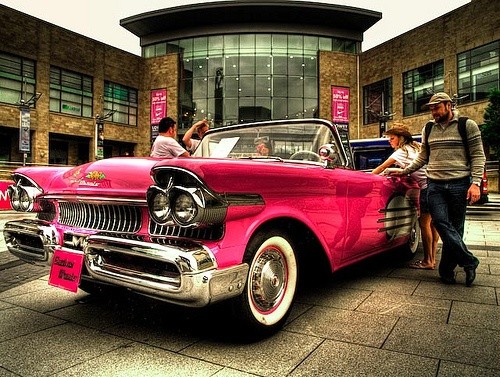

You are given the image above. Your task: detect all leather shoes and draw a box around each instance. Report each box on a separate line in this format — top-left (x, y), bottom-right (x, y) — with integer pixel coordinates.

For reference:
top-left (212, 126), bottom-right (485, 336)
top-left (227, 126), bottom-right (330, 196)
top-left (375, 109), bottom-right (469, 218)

top-left (465, 258), bottom-right (480, 286)
top-left (439, 275), bottom-right (457, 284)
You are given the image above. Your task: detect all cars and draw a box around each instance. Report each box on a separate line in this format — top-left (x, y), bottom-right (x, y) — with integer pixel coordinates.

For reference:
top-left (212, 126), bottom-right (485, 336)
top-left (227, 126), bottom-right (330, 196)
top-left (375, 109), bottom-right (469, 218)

top-left (3, 116), bottom-right (431, 336)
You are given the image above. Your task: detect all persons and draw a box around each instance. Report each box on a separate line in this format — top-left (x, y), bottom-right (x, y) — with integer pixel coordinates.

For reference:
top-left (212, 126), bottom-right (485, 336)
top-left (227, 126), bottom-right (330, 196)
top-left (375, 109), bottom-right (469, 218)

top-left (151, 117), bottom-right (192, 161)
top-left (372, 125), bottom-right (441, 270)
top-left (182, 120), bottom-right (213, 159)
top-left (382, 93), bottom-right (486, 286)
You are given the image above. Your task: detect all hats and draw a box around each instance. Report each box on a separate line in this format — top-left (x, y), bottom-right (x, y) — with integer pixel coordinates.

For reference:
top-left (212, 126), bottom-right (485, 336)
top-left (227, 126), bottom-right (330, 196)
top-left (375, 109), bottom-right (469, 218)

top-left (421, 92), bottom-right (452, 108)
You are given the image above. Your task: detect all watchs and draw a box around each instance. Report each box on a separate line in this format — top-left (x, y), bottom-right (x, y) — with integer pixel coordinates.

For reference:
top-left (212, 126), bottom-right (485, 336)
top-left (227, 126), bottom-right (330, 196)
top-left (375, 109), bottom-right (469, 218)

top-left (472, 181), bottom-right (481, 187)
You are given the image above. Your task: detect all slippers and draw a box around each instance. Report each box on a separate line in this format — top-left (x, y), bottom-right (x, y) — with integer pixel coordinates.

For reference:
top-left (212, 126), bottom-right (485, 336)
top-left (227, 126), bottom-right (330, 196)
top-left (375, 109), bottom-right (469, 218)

top-left (409, 259), bottom-right (437, 270)
